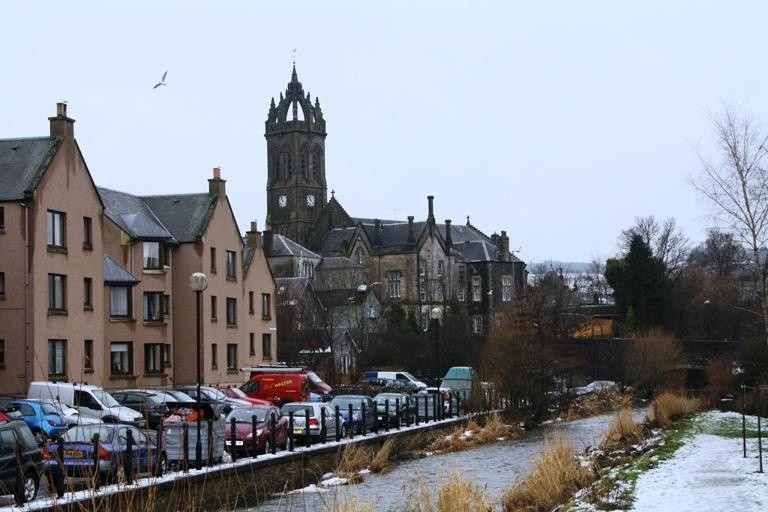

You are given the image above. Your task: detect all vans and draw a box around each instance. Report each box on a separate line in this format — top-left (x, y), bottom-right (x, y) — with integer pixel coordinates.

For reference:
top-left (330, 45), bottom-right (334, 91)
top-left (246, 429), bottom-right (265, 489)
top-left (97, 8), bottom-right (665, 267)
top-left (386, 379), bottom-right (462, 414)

top-left (364, 371), bottom-right (426, 391)
top-left (440, 366), bottom-right (483, 411)
top-left (240, 364), bottom-right (332, 403)
top-left (27, 382), bottom-right (145, 429)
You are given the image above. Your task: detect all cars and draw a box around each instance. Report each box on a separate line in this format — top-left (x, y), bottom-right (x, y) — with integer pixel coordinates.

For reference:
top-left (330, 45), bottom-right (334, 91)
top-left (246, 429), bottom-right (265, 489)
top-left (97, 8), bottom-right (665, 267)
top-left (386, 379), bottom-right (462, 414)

top-left (1, 405), bottom-right (49, 502)
top-left (111, 387), bottom-right (271, 428)
top-left (572, 381), bottom-right (613, 395)
top-left (330, 395), bottom-right (378, 435)
top-left (41, 399), bottom-right (103, 425)
top-left (2, 398), bottom-right (69, 446)
top-left (41, 423), bottom-right (166, 491)
top-left (281, 401), bottom-right (348, 443)
top-left (223, 404), bottom-right (293, 456)
top-left (373, 393), bottom-right (416, 429)
top-left (417, 388), bottom-right (464, 418)
top-left (481, 381), bottom-right (500, 409)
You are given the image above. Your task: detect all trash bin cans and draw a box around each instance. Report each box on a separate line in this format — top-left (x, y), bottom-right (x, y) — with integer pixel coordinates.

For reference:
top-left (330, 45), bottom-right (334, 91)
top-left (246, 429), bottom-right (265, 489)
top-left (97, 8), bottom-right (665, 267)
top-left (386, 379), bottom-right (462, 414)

top-left (163, 401), bottom-right (226, 466)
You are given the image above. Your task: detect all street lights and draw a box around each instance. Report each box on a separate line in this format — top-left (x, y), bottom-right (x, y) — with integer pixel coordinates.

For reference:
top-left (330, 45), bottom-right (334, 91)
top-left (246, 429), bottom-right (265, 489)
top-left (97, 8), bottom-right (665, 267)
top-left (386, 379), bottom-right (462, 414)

top-left (188, 272), bottom-right (209, 470)
top-left (431, 305), bottom-right (442, 418)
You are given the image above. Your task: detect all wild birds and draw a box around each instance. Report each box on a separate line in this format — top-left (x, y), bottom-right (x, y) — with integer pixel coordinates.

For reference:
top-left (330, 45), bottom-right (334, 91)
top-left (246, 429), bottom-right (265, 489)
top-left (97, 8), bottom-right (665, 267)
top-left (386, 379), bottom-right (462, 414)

top-left (151, 71), bottom-right (170, 91)
